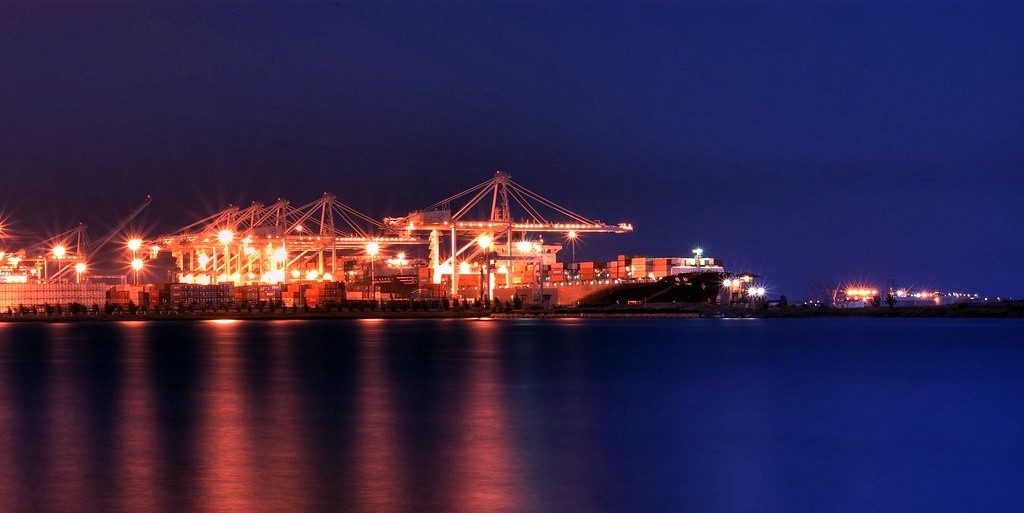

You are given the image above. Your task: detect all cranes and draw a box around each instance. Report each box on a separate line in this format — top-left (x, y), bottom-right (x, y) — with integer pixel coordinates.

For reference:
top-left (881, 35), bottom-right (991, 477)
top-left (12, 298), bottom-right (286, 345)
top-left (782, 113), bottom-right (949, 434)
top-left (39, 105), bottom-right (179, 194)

top-left (0, 168), bottom-right (634, 315)
